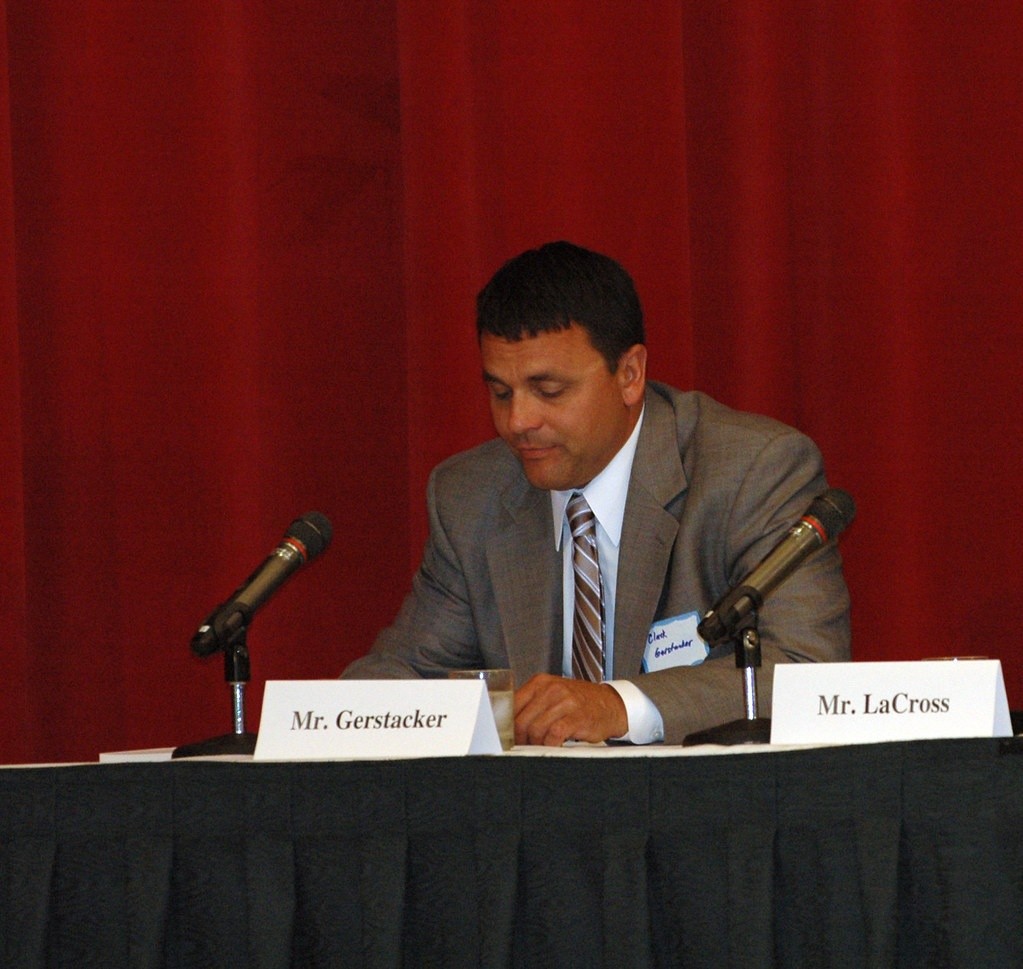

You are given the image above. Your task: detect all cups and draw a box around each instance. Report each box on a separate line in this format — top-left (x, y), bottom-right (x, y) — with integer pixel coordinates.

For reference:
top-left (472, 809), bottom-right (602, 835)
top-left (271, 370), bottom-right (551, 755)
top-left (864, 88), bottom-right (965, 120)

top-left (448, 669), bottom-right (515, 750)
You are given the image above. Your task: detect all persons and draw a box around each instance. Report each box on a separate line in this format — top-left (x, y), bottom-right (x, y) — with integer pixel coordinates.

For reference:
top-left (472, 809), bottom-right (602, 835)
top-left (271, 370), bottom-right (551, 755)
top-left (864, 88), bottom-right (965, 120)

top-left (335, 239), bottom-right (852, 751)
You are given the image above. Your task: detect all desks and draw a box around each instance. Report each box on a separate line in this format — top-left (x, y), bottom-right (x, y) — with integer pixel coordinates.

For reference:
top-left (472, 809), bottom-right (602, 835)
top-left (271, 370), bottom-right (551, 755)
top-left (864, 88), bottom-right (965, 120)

top-left (1, 735), bottom-right (1022, 969)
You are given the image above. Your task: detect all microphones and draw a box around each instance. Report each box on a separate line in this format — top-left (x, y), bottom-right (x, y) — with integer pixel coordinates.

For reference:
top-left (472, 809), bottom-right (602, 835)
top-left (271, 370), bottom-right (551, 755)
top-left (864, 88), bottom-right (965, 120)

top-left (191, 512), bottom-right (334, 658)
top-left (696, 488), bottom-right (858, 644)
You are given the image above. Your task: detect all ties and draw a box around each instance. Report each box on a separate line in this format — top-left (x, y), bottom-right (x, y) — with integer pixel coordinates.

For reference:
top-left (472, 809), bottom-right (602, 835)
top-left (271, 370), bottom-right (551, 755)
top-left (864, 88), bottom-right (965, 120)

top-left (565, 492), bottom-right (608, 685)
